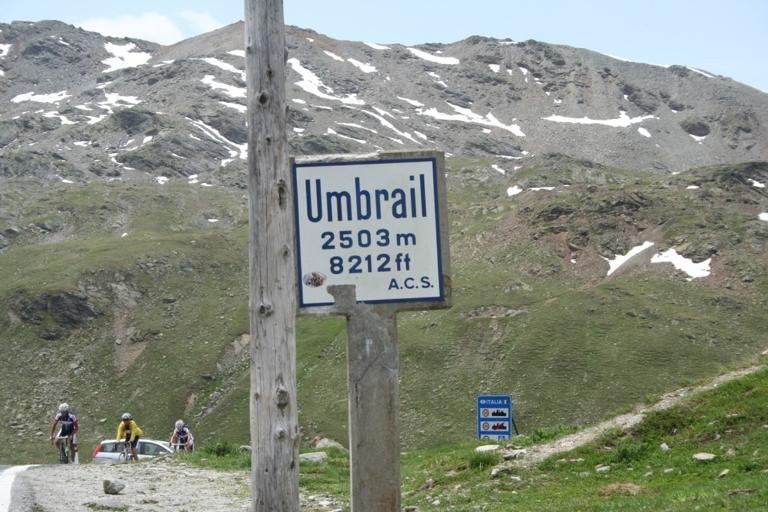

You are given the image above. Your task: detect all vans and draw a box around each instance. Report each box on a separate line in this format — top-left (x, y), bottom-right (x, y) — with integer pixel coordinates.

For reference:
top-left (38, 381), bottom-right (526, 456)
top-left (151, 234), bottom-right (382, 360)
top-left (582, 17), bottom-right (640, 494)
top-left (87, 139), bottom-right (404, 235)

top-left (91, 438), bottom-right (172, 468)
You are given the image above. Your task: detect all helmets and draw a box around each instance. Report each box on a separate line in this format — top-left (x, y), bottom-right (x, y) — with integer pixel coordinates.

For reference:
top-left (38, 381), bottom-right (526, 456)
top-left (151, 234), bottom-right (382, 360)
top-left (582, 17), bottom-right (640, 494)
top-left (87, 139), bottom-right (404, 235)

top-left (175, 419), bottom-right (183, 432)
top-left (59, 402), bottom-right (69, 412)
top-left (121, 412), bottom-right (132, 420)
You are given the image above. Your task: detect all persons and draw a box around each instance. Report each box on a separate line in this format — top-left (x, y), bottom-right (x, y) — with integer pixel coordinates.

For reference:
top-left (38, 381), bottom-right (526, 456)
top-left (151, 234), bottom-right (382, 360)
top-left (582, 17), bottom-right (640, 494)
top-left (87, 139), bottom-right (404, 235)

top-left (171, 419), bottom-right (194, 453)
top-left (115, 412), bottom-right (144, 463)
top-left (50, 403), bottom-right (79, 462)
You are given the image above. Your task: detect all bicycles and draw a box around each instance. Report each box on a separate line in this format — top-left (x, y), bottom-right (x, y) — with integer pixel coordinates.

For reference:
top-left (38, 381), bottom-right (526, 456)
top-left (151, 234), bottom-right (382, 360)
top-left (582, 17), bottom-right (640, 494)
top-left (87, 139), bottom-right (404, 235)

top-left (168, 442), bottom-right (192, 454)
top-left (50, 433), bottom-right (79, 464)
top-left (117, 440), bottom-right (144, 464)
